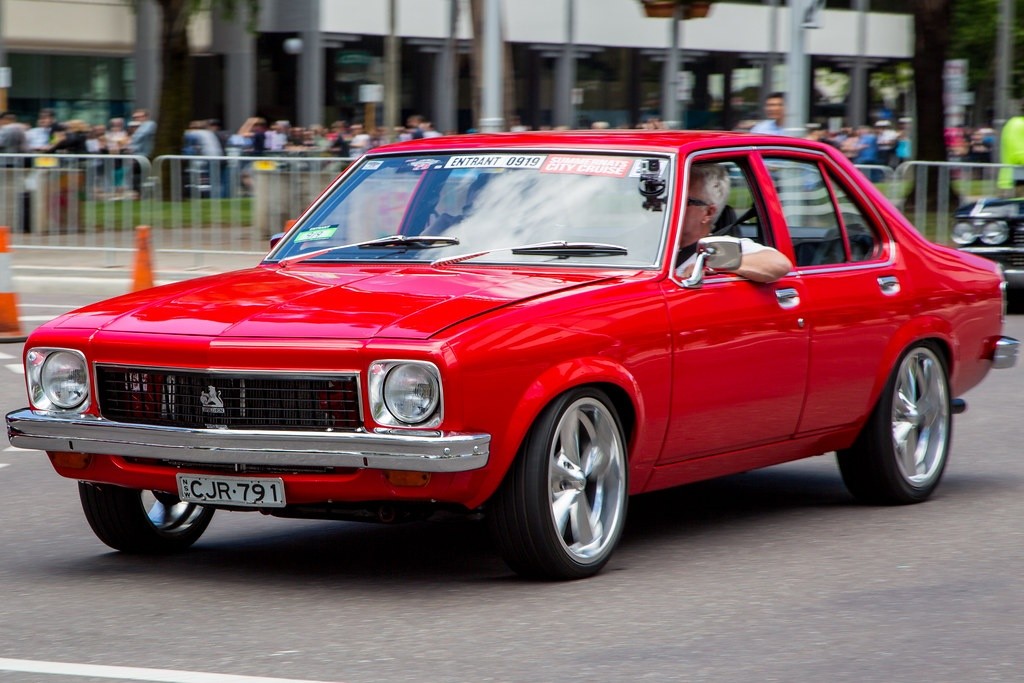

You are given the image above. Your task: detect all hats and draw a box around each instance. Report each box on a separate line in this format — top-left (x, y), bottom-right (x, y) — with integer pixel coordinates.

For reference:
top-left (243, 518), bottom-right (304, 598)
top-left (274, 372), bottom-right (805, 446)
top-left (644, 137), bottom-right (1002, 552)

top-left (898, 116), bottom-right (914, 123)
top-left (874, 119), bottom-right (892, 127)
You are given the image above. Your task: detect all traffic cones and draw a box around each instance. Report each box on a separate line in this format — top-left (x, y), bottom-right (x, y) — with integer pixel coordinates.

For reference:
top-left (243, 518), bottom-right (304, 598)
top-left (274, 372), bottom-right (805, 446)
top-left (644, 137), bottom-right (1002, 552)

top-left (1, 224), bottom-right (30, 343)
top-left (128, 224), bottom-right (155, 292)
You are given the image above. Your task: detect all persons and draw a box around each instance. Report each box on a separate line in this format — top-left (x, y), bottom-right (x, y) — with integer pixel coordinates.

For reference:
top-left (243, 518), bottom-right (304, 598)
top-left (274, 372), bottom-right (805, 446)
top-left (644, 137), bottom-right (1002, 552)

top-left (0, 92), bottom-right (1024, 233)
top-left (669, 163), bottom-right (793, 283)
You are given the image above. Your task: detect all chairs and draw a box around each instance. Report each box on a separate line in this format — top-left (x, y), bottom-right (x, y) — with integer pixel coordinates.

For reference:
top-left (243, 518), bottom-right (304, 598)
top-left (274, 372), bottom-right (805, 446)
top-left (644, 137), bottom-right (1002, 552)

top-left (712, 205), bottom-right (750, 238)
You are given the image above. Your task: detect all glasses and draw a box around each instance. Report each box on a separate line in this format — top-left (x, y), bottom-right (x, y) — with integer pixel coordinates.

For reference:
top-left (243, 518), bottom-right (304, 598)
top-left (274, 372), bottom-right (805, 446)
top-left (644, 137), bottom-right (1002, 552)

top-left (687, 198), bottom-right (711, 207)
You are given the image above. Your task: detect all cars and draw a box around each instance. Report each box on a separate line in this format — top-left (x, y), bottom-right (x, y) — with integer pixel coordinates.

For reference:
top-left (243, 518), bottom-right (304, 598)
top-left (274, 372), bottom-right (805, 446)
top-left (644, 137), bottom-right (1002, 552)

top-left (948, 193), bottom-right (1023, 303)
top-left (4, 127), bottom-right (1008, 579)
